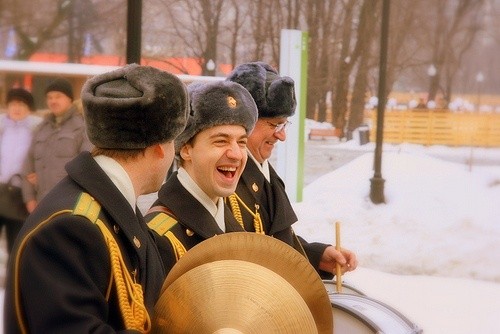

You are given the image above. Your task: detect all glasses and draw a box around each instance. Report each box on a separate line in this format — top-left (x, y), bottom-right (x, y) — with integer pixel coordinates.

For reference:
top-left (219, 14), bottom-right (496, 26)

top-left (259, 118), bottom-right (292, 133)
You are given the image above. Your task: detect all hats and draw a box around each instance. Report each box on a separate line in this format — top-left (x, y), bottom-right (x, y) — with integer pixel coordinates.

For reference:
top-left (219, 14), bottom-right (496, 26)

top-left (227, 62), bottom-right (296, 117)
top-left (6, 88), bottom-right (34, 110)
top-left (45, 76), bottom-right (74, 101)
top-left (80, 62), bottom-right (191, 151)
top-left (171, 77), bottom-right (258, 154)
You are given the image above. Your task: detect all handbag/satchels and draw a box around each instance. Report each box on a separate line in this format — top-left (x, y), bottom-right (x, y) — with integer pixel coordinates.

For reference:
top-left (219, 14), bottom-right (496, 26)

top-left (0, 173), bottom-right (31, 222)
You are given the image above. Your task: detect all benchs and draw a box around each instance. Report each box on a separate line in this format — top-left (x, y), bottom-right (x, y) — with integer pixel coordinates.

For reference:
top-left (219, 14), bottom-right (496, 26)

top-left (308, 127), bottom-right (343, 139)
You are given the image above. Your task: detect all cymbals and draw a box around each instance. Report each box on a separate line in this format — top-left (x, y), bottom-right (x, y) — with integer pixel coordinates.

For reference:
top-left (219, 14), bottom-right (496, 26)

top-left (160, 232), bottom-right (333, 334)
top-left (152, 259), bottom-right (318, 334)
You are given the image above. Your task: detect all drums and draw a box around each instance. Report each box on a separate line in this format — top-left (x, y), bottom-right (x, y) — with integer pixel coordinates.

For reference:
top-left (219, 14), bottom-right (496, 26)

top-left (322, 281), bottom-right (423, 334)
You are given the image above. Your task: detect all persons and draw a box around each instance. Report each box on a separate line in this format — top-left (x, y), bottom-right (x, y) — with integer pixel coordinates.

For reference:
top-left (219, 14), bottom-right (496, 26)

top-left (144, 81), bottom-right (259, 274)
top-left (5, 63), bottom-right (190, 334)
top-left (21, 79), bottom-right (95, 213)
top-left (1, 88), bottom-right (43, 253)
top-left (228, 62), bottom-right (357, 275)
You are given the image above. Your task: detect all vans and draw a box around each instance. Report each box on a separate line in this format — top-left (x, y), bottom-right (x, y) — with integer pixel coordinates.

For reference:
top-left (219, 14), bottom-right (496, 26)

top-left (1, 60), bottom-right (112, 116)
